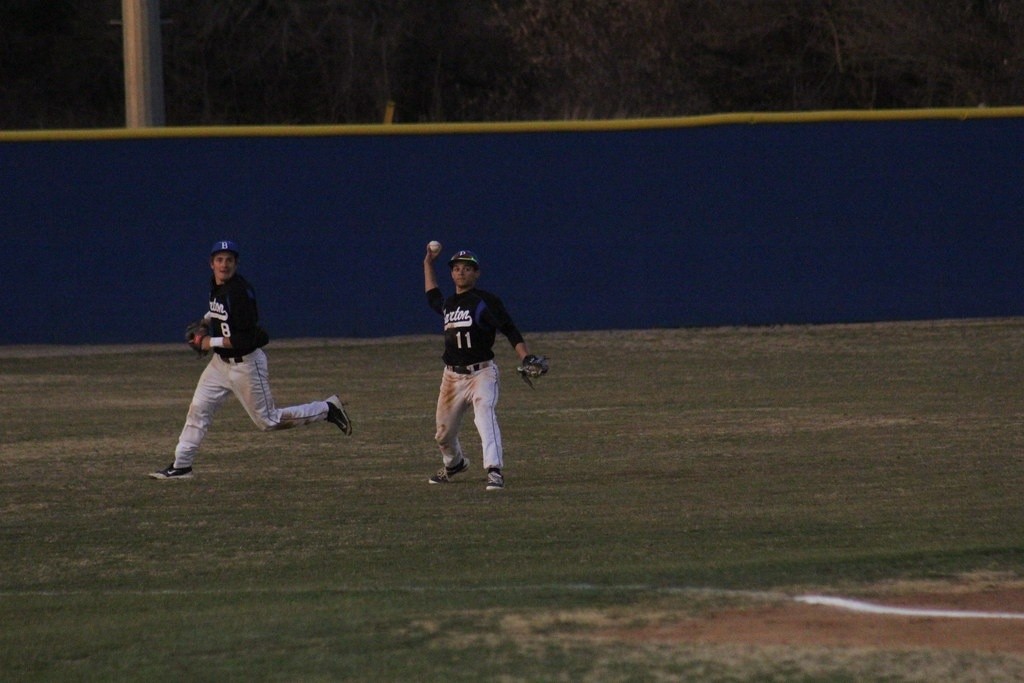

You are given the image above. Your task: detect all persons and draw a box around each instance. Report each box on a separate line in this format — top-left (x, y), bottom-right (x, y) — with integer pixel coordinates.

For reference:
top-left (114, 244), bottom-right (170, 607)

top-left (423, 241), bottom-right (550, 491)
top-left (146, 241), bottom-right (353, 478)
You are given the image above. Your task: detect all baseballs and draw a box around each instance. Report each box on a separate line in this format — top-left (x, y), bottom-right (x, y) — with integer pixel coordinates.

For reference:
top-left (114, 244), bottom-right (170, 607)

top-left (428, 240), bottom-right (441, 252)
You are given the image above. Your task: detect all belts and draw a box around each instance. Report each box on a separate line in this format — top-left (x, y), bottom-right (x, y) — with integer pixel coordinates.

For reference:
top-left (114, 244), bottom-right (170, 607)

top-left (447, 364), bottom-right (479, 374)
top-left (220, 355), bottom-right (243, 363)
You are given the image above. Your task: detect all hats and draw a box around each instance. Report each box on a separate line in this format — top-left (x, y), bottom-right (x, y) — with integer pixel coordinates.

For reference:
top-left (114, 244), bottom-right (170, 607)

top-left (448, 250), bottom-right (479, 268)
top-left (211, 241), bottom-right (239, 256)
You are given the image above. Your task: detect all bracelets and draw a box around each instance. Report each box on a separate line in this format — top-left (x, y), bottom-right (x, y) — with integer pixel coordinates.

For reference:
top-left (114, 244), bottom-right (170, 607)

top-left (209, 337), bottom-right (224, 348)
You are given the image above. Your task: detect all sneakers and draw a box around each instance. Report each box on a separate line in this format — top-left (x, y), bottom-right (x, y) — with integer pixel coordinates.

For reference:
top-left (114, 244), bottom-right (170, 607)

top-left (485, 471), bottom-right (504, 491)
top-left (325, 394), bottom-right (352, 436)
top-left (149, 463), bottom-right (193, 479)
top-left (429, 458), bottom-right (470, 483)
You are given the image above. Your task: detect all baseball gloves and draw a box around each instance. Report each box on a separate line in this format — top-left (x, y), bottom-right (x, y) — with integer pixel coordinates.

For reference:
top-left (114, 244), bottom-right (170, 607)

top-left (516, 354), bottom-right (550, 379)
top-left (184, 318), bottom-right (213, 354)
